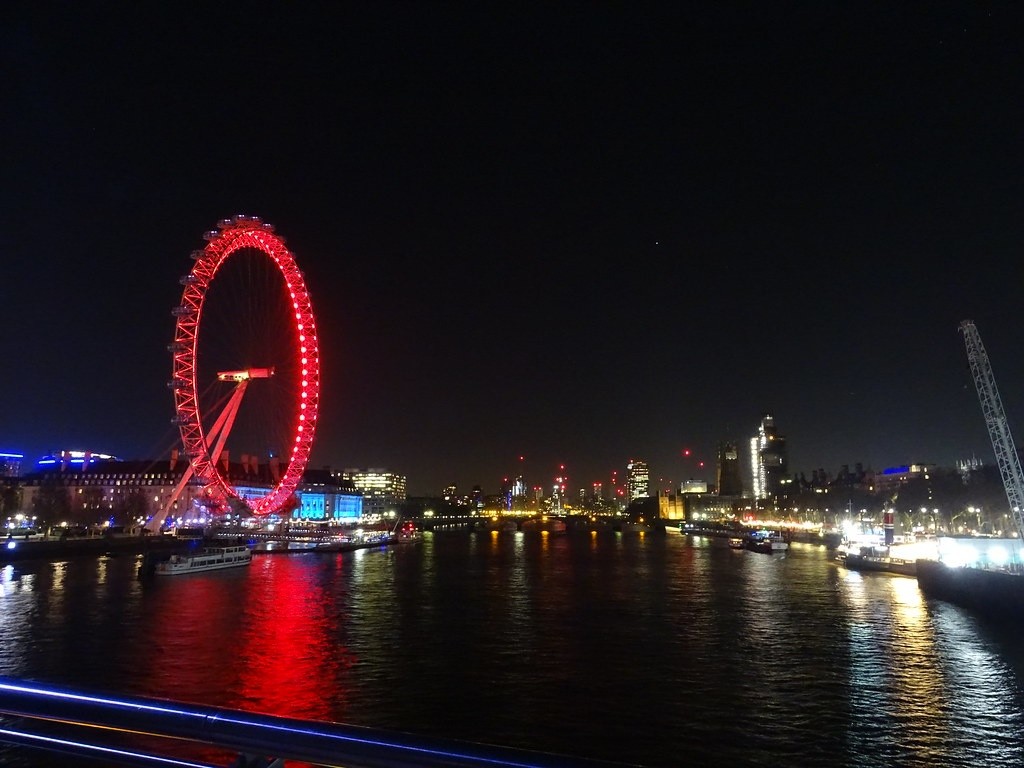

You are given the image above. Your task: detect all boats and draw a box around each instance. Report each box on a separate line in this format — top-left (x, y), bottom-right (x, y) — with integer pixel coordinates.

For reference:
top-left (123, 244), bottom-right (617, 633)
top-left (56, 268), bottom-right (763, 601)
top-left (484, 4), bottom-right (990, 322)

top-left (842, 543), bottom-right (891, 572)
top-left (725, 531), bottom-right (791, 555)
top-left (153, 545), bottom-right (253, 575)
